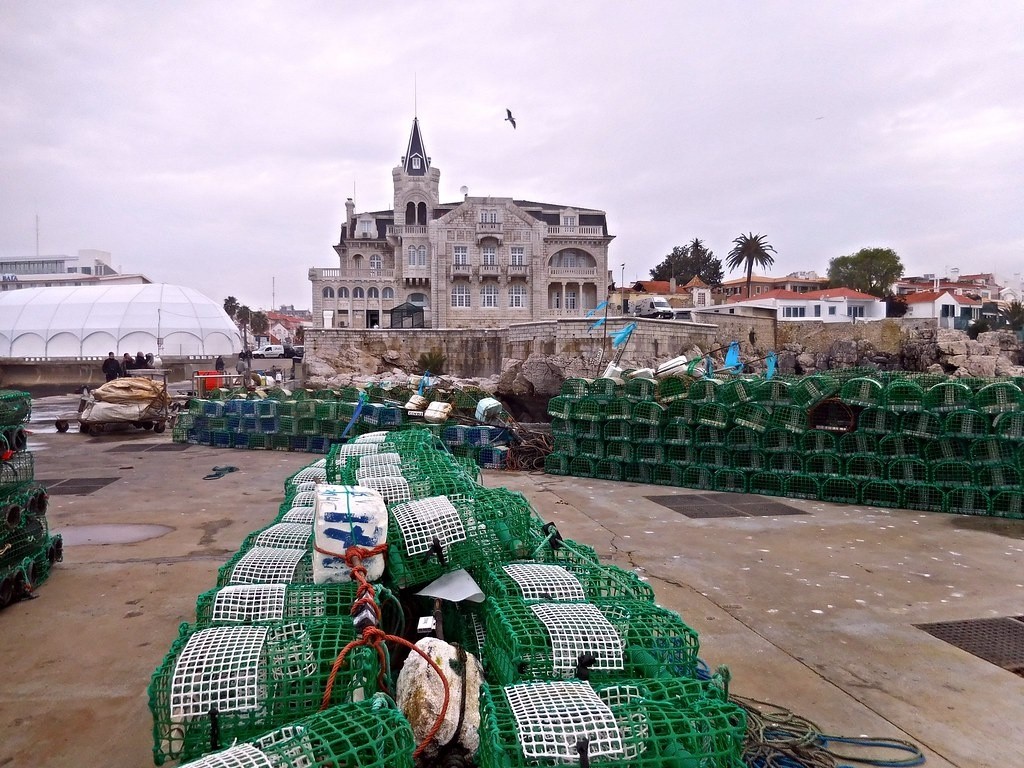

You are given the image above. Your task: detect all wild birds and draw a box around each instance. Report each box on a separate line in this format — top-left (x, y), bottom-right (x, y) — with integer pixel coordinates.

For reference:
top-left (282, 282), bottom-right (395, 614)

top-left (505, 109), bottom-right (518, 129)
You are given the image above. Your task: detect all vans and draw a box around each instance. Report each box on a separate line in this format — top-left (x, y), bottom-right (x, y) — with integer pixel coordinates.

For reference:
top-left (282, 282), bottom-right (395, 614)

top-left (634, 296), bottom-right (674, 319)
top-left (251, 344), bottom-right (285, 360)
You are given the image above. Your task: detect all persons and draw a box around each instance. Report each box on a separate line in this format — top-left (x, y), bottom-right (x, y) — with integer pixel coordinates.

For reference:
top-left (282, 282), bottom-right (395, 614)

top-left (246, 350), bottom-right (252, 360)
top-left (102, 351), bottom-right (122, 382)
top-left (135, 351), bottom-right (155, 369)
top-left (216, 355), bottom-right (225, 370)
top-left (119, 353), bottom-right (134, 377)
top-left (236, 358), bottom-right (246, 375)
top-left (239, 350), bottom-right (245, 360)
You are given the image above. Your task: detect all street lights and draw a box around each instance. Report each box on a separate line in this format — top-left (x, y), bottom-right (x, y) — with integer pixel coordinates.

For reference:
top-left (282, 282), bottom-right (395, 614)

top-left (621, 263), bottom-right (625, 316)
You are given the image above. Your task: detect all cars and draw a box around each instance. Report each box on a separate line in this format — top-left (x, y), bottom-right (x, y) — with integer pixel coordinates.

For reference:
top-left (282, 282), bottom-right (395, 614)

top-left (282, 345), bottom-right (304, 359)
